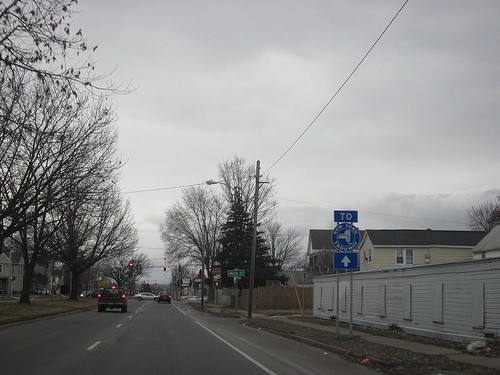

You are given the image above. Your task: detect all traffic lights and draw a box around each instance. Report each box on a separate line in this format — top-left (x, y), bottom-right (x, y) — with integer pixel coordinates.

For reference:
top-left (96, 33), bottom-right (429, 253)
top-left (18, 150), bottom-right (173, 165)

top-left (164, 265), bottom-right (166, 271)
top-left (129, 262), bottom-right (133, 270)
top-left (12, 275), bottom-right (16, 281)
top-left (136, 264), bottom-right (138, 270)
top-left (140, 264), bottom-right (142, 271)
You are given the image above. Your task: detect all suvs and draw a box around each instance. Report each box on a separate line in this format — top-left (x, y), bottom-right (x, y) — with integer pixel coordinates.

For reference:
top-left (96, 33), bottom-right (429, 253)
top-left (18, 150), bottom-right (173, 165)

top-left (97, 287), bottom-right (128, 313)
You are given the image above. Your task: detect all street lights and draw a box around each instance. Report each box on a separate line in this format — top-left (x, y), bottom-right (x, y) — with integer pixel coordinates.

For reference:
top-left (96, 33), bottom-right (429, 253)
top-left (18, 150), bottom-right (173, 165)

top-left (204, 179), bottom-right (254, 321)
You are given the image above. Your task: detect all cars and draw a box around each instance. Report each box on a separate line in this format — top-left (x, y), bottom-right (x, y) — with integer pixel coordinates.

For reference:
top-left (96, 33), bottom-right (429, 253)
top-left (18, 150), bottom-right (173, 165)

top-left (81, 289), bottom-right (99, 299)
top-left (135, 292), bottom-right (159, 302)
top-left (157, 292), bottom-right (172, 304)
